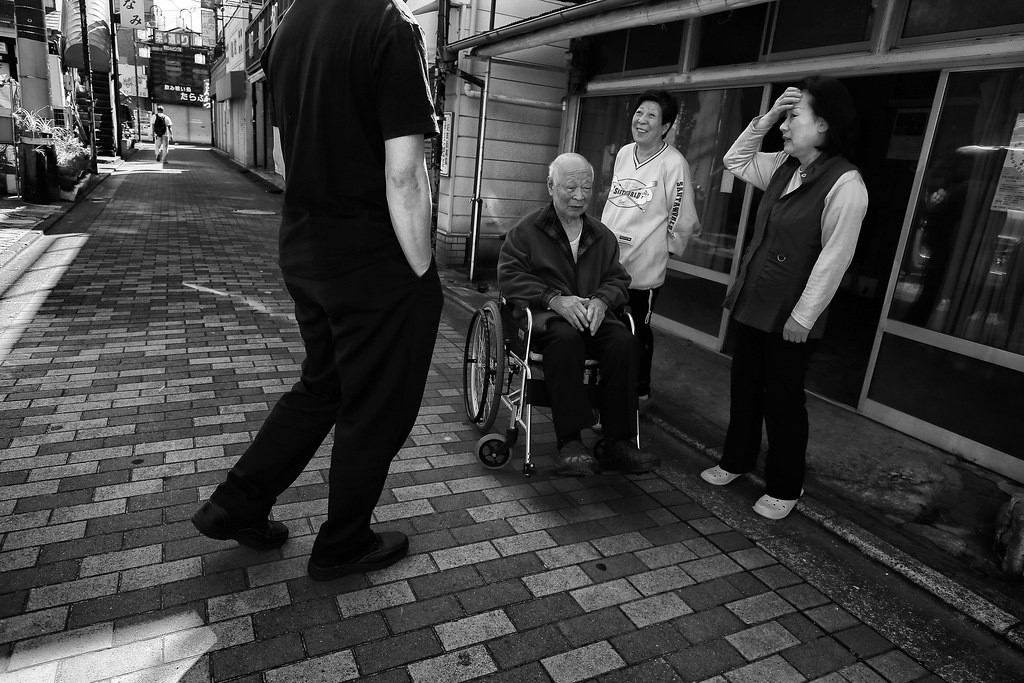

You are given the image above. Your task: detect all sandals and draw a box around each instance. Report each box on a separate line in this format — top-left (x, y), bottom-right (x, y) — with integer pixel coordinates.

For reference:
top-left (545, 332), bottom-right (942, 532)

top-left (752, 488), bottom-right (804, 519)
top-left (701, 465), bottom-right (743, 485)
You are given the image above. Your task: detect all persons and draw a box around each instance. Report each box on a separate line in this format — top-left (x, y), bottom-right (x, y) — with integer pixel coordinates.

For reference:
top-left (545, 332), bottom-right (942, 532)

top-left (700, 76), bottom-right (868, 519)
top-left (192, 1), bottom-right (445, 580)
top-left (602, 89), bottom-right (702, 416)
top-left (497, 152), bottom-right (652, 476)
top-left (151, 106), bottom-right (173, 163)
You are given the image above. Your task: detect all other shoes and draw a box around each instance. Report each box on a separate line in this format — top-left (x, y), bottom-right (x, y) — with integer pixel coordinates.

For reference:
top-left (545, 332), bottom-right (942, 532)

top-left (603, 439), bottom-right (660, 467)
top-left (155, 153), bottom-right (160, 162)
top-left (163, 162), bottom-right (168, 164)
top-left (557, 440), bottom-right (599, 478)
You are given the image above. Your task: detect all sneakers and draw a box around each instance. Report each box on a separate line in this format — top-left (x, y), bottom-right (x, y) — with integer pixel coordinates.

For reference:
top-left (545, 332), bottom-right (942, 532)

top-left (191, 498), bottom-right (288, 550)
top-left (307, 528), bottom-right (409, 583)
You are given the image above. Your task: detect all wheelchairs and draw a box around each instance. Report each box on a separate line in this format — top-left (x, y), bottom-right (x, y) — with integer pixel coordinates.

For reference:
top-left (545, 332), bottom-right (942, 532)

top-left (462, 232), bottom-right (642, 480)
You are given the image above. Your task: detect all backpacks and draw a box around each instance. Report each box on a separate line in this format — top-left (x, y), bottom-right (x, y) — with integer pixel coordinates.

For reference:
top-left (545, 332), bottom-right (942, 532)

top-left (154, 114), bottom-right (167, 137)
